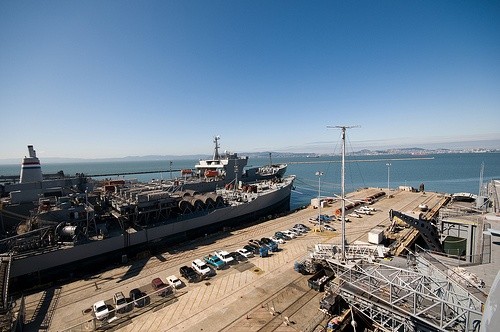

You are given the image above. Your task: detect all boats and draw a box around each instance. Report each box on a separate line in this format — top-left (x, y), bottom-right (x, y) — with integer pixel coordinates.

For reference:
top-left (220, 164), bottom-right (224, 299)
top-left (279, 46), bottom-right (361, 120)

top-left (0, 134), bottom-right (296, 280)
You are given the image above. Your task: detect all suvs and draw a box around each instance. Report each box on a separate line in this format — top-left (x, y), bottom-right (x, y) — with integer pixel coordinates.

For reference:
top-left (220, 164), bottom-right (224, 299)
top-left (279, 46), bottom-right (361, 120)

top-left (113, 291), bottom-right (128, 314)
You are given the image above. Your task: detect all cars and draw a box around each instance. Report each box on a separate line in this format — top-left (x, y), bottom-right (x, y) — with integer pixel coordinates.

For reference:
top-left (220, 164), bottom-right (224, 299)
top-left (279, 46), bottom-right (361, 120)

top-left (166, 275), bottom-right (183, 289)
top-left (151, 277), bottom-right (168, 297)
top-left (128, 289), bottom-right (145, 309)
top-left (92, 300), bottom-right (110, 320)
top-left (180, 205), bottom-right (377, 284)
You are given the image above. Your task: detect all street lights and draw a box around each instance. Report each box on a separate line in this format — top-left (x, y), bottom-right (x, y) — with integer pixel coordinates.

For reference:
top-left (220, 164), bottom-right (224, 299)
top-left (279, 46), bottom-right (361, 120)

top-left (314, 171), bottom-right (324, 228)
top-left (386, 163), bottom-right (393, 197)
